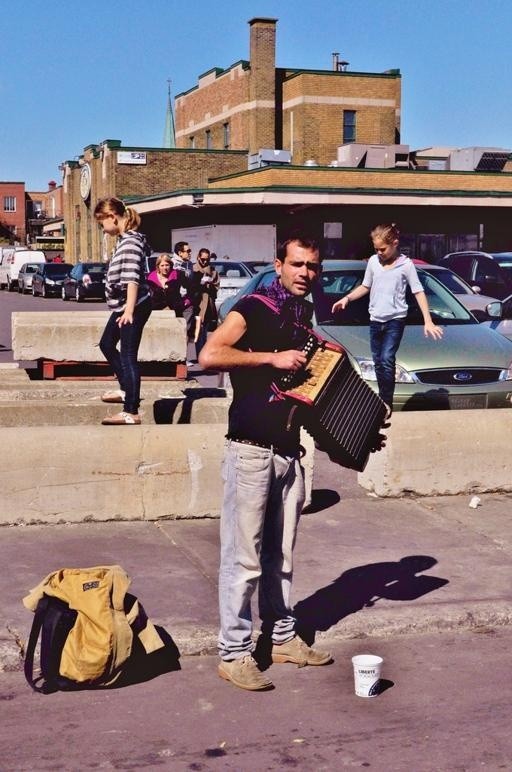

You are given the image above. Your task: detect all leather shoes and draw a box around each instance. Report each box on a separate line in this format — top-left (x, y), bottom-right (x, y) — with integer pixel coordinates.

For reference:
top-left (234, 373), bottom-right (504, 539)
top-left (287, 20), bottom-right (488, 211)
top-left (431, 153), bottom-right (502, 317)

top-left (101, 389), bottom-right (126, 403)
top-left (100, 412), bottom-right (143, 424)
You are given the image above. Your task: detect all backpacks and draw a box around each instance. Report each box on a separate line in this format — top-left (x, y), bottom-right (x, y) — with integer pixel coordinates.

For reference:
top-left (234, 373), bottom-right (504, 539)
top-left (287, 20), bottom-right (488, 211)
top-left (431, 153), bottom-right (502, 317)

top-left (21, 562), bottom-right (166, 695)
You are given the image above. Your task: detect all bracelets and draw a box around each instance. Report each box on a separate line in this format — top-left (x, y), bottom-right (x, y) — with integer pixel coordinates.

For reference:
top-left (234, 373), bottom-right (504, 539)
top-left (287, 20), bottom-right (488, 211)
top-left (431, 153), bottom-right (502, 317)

top-left (344, 295), bottom-right (351, 305)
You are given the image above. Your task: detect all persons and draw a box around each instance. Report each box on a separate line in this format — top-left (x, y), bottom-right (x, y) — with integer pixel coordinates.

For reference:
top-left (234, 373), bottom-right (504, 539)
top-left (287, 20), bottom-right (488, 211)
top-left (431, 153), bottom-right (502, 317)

top-left (146, 241), bottom-right (230, 368)
top-left (91, 195), bottom-right (153, 425)
top-left (331, 221), bottom-right (444, 418)
top-left (197, 235), bottom-right (334, 693)
top-left (52, 254), bottom-right (61, 264)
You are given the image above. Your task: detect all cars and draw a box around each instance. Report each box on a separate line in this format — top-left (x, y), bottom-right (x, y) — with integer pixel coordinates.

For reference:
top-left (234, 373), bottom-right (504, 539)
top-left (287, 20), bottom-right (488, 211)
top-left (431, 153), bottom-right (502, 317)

top-left (218, 257), bottom-right (511, 408)
top-left (15, 262), bottom-right (112, 302)
top-left (208, 260), bottom-right (270, 315)
top-left (415, 249), bottom-right (511, 352)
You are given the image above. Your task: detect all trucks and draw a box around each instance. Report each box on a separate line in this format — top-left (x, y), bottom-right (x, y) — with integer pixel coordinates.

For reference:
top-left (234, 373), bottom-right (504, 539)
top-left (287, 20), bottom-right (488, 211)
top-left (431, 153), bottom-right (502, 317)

top-left (0, 249), bottom-right (47, 290)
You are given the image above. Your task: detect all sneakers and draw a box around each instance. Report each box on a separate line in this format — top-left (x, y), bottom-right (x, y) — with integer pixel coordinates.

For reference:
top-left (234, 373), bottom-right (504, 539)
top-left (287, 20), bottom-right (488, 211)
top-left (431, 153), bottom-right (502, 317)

top-left (216, 655), bottom-right (274, 693)
top-left (269, 635), bottom-right (332, 666)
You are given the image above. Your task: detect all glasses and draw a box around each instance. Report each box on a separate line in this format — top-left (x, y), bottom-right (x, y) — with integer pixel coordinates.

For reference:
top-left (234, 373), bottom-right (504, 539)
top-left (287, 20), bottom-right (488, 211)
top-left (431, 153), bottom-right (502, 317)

top-left (200, 257), bottom-right (211, 262)
top-left (181, 248), bottom-right (192, 253)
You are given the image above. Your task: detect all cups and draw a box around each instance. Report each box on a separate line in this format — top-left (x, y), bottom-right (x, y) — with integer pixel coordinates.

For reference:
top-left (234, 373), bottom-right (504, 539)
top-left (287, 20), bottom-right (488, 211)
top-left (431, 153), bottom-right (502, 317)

top-left (352, 654), bottom-right (384, 699)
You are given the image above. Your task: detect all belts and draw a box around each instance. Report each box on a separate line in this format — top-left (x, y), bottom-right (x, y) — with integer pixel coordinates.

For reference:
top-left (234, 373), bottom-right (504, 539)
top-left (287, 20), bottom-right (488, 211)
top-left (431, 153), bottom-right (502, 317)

top-left (226, 438), bottom-right (301, 453)
